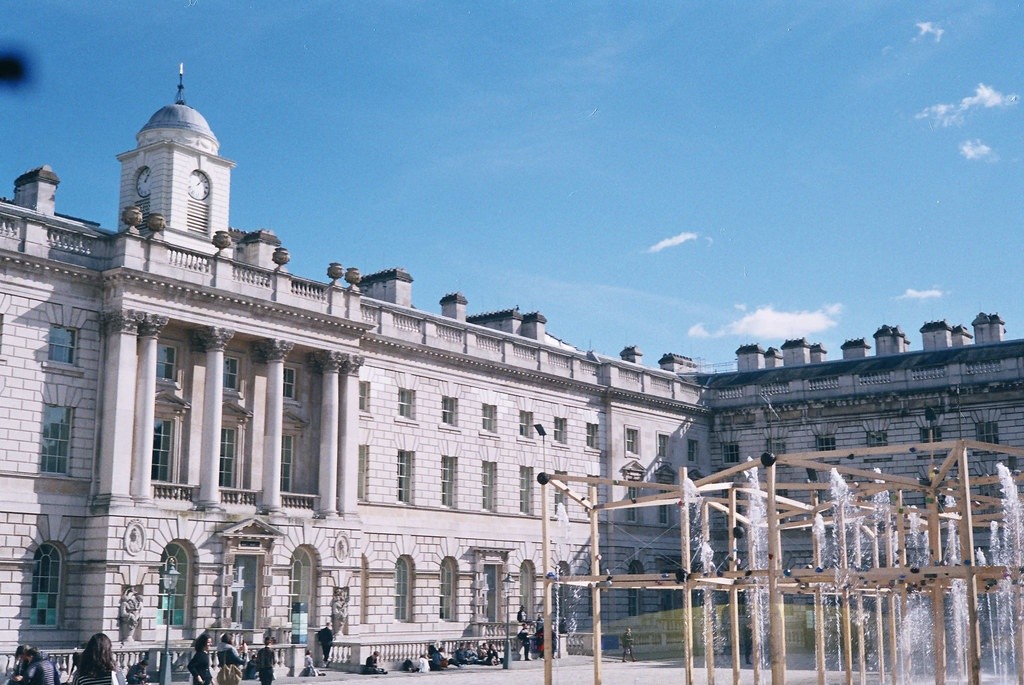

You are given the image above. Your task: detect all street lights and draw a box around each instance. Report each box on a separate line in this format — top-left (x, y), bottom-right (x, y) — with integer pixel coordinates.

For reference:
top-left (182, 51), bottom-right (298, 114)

top-left (501, 571), bottom-right (516, 669)
top-left (159, 555), bottom-right (180, 685)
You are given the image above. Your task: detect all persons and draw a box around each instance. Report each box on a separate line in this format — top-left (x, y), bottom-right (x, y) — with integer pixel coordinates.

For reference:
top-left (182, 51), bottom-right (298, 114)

top-left (318, 621), bottom-right (333, 668)
top-left (365, 650), bottom-right (388, 674)
top-left (4, 632), bottom-right (278, 685)
top-left (622, 627), bottom-right (637, 663)
top-left (517, 605), bottom-right (568, 661)
top-left (743, 622), bottom-right (753, 665)
top-left (409, 642), bottom-right (505, 674)
top-left (304, 650), bottom-right (326, 677)
